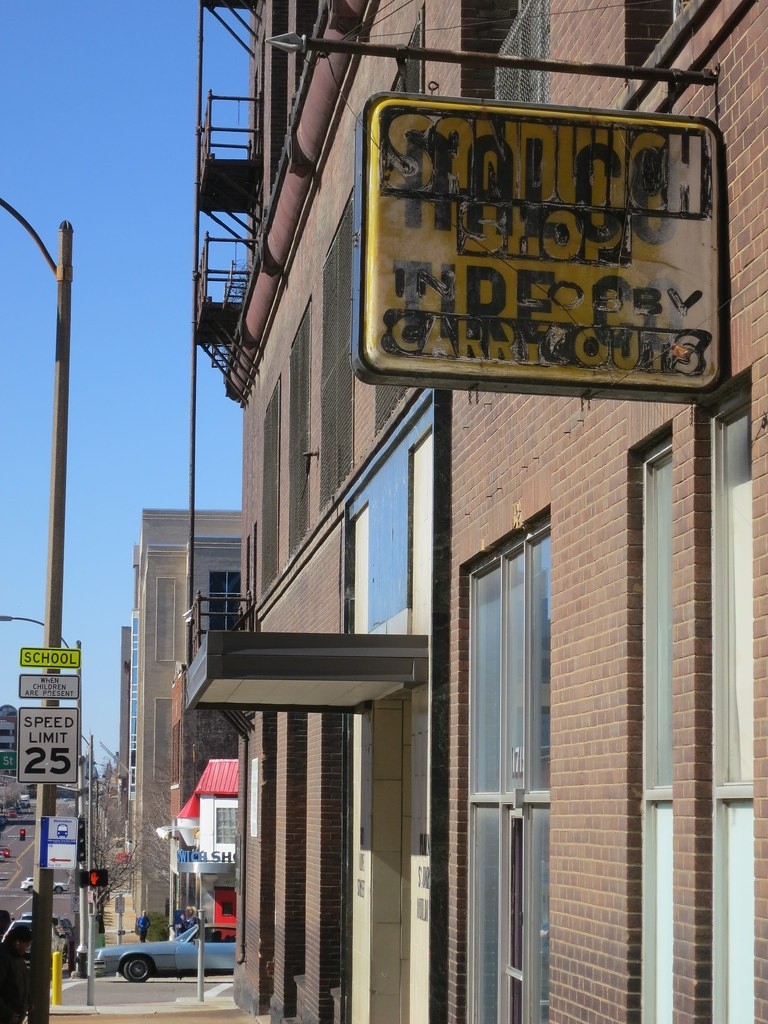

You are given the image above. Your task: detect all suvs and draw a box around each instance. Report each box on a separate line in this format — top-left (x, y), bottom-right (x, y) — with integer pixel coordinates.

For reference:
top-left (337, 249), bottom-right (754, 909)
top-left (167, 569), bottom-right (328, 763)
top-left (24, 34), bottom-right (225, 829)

top-left (18, 912), bottom-right (73, 947)
top-left (1, 919), bottom-right (67, 969)
top-left (20, 877), bottom-right (69, 893)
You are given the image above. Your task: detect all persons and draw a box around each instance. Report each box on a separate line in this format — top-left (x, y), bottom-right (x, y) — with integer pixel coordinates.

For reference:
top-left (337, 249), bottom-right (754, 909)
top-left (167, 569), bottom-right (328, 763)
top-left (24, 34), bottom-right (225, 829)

top-left (179, 906), bottom-right (196, 934)
top-left (137, 910), bottom-right (150, 943)
top-left (0, 909), bottom-right (31, 1024)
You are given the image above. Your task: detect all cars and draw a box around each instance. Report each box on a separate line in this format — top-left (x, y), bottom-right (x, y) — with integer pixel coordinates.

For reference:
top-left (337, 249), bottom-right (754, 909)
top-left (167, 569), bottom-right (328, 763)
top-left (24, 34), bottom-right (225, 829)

top-left (0, 847), bottom-right (10, 862)
top-left (94, 923), bottom-right (238, 983)
top-left (7, 805), bottom-right (19, 818)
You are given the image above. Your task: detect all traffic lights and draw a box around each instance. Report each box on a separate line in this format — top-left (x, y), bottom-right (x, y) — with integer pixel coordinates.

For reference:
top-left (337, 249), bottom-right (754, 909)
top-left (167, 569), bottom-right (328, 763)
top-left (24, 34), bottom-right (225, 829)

top-left (90, 869), bottom-right (108, 887)
top-left (20, 828), bottom-right (25, 841)
top-left (78, 817), bottom-right (87, 861)
top-left (80, 869), bottom-right (90, 888)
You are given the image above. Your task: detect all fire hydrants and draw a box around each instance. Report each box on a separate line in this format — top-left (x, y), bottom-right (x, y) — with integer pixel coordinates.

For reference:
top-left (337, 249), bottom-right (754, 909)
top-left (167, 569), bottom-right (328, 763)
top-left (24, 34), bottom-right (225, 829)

top-left (74, 953), bottom-right (87, 979)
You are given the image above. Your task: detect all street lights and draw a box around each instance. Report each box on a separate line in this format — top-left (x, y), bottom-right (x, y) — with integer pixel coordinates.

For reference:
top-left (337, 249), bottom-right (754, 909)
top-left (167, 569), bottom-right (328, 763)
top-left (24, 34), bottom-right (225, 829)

top-left (0, 615), bottom-right (87, 952)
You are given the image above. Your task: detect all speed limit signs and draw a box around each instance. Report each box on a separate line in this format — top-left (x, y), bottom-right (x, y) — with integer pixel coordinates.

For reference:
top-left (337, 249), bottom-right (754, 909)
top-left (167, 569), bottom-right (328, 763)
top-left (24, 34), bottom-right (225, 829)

top-left (16, 706), bottom-right (79, 784)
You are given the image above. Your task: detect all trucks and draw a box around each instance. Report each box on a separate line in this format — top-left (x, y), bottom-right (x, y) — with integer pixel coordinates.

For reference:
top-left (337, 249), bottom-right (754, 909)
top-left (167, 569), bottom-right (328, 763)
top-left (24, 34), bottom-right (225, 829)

top-left (20, 794), bottom-right (30, 806)
